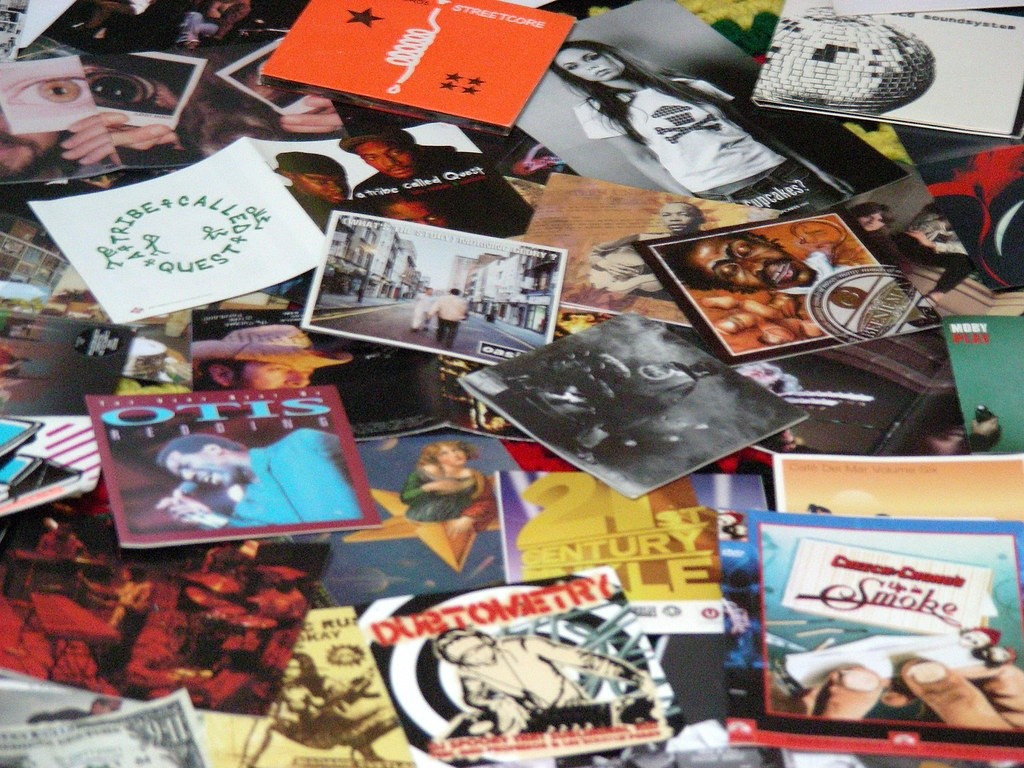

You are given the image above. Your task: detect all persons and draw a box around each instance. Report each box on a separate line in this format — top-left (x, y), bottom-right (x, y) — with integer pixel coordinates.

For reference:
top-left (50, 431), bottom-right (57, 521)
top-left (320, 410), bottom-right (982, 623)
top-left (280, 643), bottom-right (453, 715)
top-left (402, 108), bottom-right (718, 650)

top-left (0, 0), bottom-right (1024, 768)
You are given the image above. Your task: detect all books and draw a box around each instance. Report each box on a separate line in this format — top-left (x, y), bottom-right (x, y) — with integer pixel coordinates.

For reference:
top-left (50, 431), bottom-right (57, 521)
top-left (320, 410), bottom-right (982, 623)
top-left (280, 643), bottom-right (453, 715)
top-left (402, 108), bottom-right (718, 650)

top-left (257, 1), bottom-right (575, 136)
top-left (86, 385), bottom-right (384, 550)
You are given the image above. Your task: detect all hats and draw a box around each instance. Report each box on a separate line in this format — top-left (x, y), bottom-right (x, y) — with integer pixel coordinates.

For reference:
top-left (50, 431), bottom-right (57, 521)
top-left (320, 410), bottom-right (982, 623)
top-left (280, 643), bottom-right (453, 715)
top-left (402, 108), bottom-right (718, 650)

top-left (273, 152), bottom-right (346, 176)
top-left (338, 126), bottom-right (413, 153)
